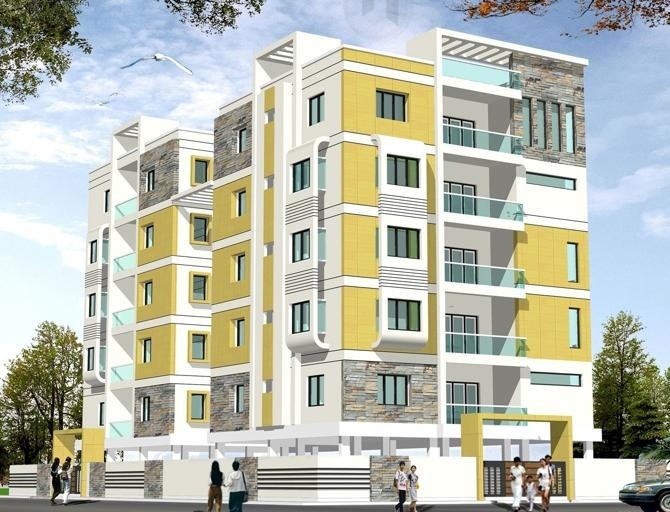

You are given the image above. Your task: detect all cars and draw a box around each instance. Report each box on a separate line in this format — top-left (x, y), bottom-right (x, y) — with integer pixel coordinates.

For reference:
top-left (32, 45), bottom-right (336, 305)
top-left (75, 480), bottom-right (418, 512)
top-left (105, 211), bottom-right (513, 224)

top-left (619, 460), bottom-right (670, 511)
